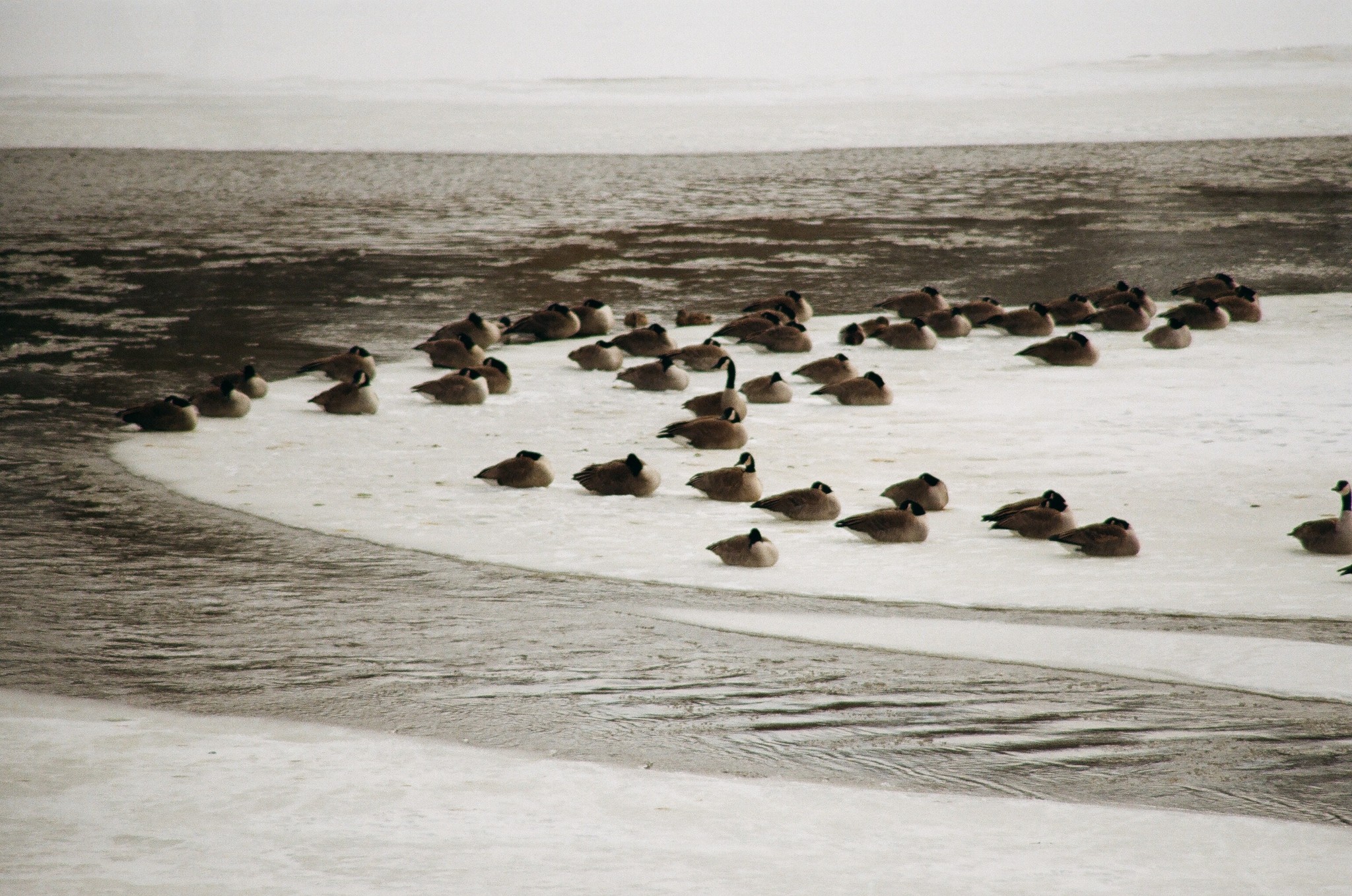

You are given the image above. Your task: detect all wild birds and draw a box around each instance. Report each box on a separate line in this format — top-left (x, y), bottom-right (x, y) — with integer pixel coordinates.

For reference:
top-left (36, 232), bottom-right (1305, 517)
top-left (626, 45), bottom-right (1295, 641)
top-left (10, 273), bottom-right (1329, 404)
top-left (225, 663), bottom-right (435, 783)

top-left (409, 356), bottom-right (513, 405)
top-left (1285, 479), bottom-right (1352, 578)
top-left (1050, 514), bottom-right (1149, 564)
top-left (412, 297), bottom-right (617, 370)
top-left (295, 344), bottom-right (377, 385)
top-left (473, 449), bottom-right (555, 489)
top-left (704, 527), bottom-right (780, 568)
top-left (981, 487), bottom-right (1079, 542)
top-left (306, 369), bottom-right (380, 416)
top-left (596, 272), bottom-right (1262, 520)
top-left (190, 363), bottom-right (269, 419)
top-left (115, 396), bottom-right (199, 433)
top-left (572, 451), bottom-right (662, 497)
top-left (834, 500), bottom-right (929, 544)
